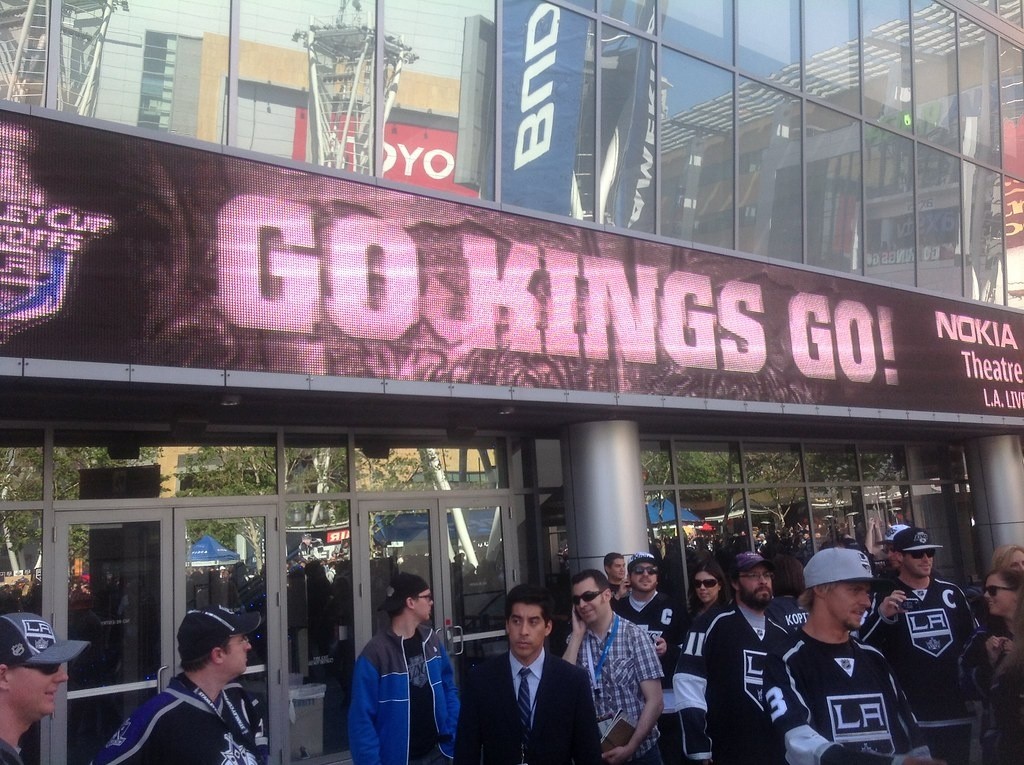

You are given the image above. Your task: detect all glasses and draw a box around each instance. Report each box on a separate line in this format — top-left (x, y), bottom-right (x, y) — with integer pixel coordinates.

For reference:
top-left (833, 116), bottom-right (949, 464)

top-left (38, 663), bottom-right (61, 674)
top-left (741, 571), bottom-right (775, 580)
top-left (910, 548), bottom-right (935, 558)
top-left (418, 592), bottom-right (432, 600)
top-left (984, 585), bottom-right (1014, 595)
top-left (692, 579), bottom-right (719, 589)
top-left (225, 634), bottom-right (249, 645)
top-left (573, 586), bottom-right (607, 605)
top-left (633, 566), bottom-right (658, 574)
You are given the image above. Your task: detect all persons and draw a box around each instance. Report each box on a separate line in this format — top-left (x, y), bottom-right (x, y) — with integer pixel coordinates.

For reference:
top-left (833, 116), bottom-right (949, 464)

top-left (191, 542), bottom-right (349, 587)
top-left (89, 605), bottom-right (273, 765)
top-left (453, 584), bottom-right (606, 765)
top-left (6, 573), bottom-right (90, 596)
top-left (347, 572), bottom-right (458, 765)
top-left (0, 611), bottom-right (89, 765)
top-left (651, 520), bottom-right (844, 559)
top-left (560, 524), bottom-right (1024, 765)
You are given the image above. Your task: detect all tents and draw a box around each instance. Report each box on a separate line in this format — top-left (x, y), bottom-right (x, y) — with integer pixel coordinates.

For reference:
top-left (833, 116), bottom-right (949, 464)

top-left (185, 534), bottom-right (241, 567)
top-left (644, 496), bottom-right (700, 526)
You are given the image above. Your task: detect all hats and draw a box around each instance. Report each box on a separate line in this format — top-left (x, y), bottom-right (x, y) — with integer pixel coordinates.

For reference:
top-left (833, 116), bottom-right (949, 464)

top-left (804, 546), bottom-right (895, 594)
top-left (894, 526), bottom-right (944, 551)
top-left (628, 550), bottom-right (656, 574)
top-left (177, 605), bottom-right (261, 662)
top-left (0, 612), bottom-right (91, 666)
top-left (377, 572), bottom-right (429, 611)
top-left (728, 552), bottom-right (775, 580)
top-left (875, 524), bottom-right (911, 544)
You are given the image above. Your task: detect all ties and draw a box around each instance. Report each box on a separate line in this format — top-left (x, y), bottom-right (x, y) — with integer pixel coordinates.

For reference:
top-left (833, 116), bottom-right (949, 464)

top-left (518, 668), bottom-right (532, 752)
